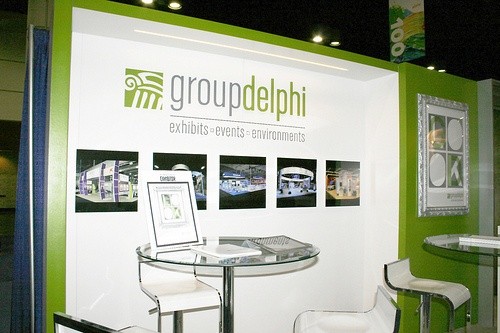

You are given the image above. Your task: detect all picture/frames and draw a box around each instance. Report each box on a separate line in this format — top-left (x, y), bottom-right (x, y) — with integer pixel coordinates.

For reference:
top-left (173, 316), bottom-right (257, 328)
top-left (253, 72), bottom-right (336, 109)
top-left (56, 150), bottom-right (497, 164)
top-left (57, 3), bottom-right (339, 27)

top-left (418, 93), bottom-right (470, 216)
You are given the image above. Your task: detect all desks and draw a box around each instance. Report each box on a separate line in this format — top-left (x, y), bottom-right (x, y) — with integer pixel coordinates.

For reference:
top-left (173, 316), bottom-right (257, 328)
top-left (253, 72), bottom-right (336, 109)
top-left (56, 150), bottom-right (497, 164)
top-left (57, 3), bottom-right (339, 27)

top-left (136, 236), bottom-right (320, 333)
top-left (424, 234), bottom-right (500, 333)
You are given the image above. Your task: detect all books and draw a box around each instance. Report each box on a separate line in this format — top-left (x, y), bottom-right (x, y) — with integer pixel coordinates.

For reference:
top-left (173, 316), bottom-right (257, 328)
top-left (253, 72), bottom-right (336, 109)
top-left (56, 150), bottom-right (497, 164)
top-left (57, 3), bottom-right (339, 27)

top-left (248, 235), bottom-right (313, 254)
top-left (459, 234), bottom-right (500, 249)
top-left (191, 244), bottom-right (262, 259)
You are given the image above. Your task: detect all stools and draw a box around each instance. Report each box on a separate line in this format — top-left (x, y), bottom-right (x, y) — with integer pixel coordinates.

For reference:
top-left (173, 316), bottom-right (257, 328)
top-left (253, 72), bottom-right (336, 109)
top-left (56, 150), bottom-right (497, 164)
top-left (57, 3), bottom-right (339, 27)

top-left (294, 286), bottom-right (401, 333)
top-left (53, 312), bottom-right (161, 333)
top-left (384, 258), bottom-right (471, 333)
top-left (139, 260), bottom-right (222, 333)
